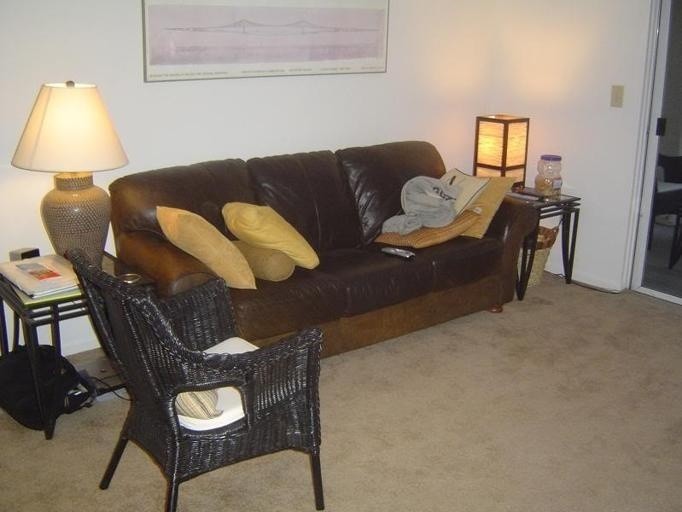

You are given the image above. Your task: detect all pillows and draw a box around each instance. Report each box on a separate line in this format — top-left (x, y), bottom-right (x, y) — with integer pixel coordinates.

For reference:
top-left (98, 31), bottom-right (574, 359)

top-left (152, 198), bottom-right (321, 291)
top-left (172, 382), bottom-right (225, 420)
top-left (370, 166), bottom-right (516, 249)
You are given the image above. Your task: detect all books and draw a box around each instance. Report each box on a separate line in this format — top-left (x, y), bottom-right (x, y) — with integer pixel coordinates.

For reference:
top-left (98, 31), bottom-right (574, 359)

top-left (0, 254), bottom-right (81, 306)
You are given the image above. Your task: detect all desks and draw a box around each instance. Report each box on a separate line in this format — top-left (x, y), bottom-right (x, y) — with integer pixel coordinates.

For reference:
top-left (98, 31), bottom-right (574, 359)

top-left (0, 250), bottom-right (159, 440)
top-left (506, 187), bottom-right (582, 301)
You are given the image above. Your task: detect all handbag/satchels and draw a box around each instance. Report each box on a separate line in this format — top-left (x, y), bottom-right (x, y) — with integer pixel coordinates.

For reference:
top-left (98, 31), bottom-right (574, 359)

top-left (0, 344), bottom-right (95, 431)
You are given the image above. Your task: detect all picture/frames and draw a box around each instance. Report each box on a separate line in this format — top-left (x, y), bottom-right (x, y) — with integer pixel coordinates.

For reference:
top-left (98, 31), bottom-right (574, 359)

top-left (140, 0), bottom-right (392, 83)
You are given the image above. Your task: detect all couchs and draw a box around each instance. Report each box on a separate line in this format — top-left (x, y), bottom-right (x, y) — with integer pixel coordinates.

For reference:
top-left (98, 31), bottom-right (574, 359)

top-left (107, 140), bottom-right (541, 362)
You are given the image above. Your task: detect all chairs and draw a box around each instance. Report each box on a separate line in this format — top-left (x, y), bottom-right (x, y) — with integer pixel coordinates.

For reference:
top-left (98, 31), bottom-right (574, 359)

top-left (67, 246), bottom-right (327, 512)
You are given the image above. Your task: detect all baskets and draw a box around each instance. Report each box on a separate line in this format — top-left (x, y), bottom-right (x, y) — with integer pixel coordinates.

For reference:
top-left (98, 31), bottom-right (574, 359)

top-left (517, 226), bottom-right (558, 288)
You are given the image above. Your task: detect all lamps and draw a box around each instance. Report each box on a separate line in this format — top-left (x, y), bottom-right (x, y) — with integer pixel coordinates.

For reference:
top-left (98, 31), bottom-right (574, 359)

top-left (471, 112), bottom-right (529, 192)
top-left (8, 79), bottom-right (131, 276)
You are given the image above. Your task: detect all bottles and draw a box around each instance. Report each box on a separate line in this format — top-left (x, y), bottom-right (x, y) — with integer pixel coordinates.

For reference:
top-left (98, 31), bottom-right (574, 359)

top-left (535, 154), bottom-right (563, 197)
